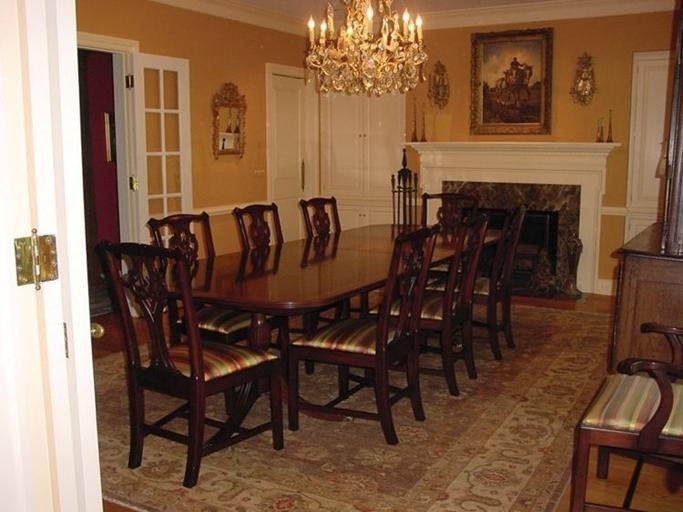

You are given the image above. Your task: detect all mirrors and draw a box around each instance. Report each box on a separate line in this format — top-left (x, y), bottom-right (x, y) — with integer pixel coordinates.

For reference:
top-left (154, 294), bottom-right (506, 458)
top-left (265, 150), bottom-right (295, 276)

top-left (211, 82), bottom-right (247, 159)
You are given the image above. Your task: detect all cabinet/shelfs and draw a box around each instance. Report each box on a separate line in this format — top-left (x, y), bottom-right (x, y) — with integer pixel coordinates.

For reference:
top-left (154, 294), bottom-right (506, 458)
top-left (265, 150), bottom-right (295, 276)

top-left (659, 4), bottom-right (683, 259)
top-left (607, 222), bottom-right (683, 375)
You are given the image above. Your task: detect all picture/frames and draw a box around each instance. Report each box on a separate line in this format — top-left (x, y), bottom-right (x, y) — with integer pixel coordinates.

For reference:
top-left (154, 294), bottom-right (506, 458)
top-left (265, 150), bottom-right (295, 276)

top-left (469, 27), bottom-right (553, 135)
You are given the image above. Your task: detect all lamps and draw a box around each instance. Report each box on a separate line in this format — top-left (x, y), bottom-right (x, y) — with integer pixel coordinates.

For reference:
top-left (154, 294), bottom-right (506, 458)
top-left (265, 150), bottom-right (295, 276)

top-left (304, 1), bottom-right (428, 98)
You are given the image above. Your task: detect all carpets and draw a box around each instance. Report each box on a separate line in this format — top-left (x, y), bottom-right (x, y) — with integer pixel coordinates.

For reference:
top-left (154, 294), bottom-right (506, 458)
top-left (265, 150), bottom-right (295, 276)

top-left (94, 301), bottom-right (614, 512)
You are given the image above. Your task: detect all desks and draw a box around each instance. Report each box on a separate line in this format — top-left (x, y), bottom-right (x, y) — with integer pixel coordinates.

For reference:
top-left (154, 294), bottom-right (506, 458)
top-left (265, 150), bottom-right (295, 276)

top-left (116, 223), bottom-right (512, 323)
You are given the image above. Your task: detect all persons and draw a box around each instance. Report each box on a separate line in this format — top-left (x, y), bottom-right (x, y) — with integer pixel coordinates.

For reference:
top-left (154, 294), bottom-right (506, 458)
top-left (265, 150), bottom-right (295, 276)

top-left (510, 55), bottom-right (525, 79)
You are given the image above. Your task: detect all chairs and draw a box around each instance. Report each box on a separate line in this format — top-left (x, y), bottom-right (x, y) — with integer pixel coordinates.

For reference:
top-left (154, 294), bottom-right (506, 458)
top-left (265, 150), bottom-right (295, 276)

top-left (292, 222), bottom-right (441, 445)
top-left (95, 239), bottom-right (286, 489)
top-left (297, 197), bottom-right (343, 234)
top-left (427, 201), bottom-right (527, 362)
top-left (231, 201), bottom-right (285, 248)
top-left (147, 209), bottom-right (281, 348)
top-left (370, 209), bottom-right (495, 399)
top-left (570, 322), bottom-right (683, 512)
